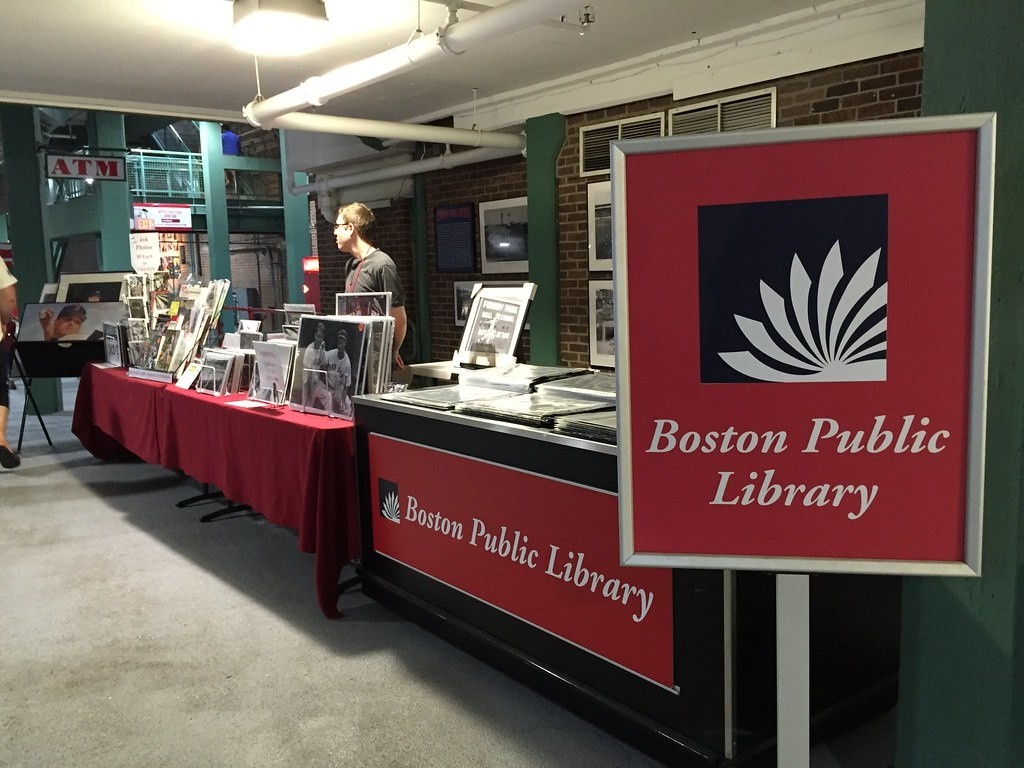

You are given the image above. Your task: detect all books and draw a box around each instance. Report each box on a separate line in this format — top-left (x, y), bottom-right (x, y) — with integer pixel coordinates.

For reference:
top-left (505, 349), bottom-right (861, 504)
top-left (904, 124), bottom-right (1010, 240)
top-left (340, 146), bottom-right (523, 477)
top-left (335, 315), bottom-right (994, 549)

top-left (251, 304), bottom-right (317, 401)
top-left (174, 319), bottom-right (263, 397)
top-left (141, 278), bottom-right (230, 374)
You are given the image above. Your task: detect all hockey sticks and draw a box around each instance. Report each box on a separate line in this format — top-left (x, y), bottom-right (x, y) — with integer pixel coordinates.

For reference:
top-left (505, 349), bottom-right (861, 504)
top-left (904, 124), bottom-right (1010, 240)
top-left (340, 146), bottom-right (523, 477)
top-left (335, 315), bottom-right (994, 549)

top-left (340, 374), bottom-right (348, 415)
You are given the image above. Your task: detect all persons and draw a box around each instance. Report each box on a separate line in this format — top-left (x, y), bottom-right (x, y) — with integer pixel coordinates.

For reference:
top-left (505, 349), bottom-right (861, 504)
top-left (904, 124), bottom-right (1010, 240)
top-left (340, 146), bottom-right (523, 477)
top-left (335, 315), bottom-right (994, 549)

top-left (333, 201), bottom-right (409, 371)
top-left (39, 304), bottom-right (87, 340)
top-left (303, 323), bottom-right (352, 415)
top-left (0, 254), bottom-right (21, 469)
top-left (222, 124), bottom-right (240, 193)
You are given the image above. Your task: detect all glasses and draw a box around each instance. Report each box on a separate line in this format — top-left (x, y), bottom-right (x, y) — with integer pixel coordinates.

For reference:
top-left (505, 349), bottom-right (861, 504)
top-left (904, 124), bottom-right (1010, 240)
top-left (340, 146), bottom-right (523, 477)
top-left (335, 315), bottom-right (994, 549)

top-left (331, 223), bottom-right (349, 230)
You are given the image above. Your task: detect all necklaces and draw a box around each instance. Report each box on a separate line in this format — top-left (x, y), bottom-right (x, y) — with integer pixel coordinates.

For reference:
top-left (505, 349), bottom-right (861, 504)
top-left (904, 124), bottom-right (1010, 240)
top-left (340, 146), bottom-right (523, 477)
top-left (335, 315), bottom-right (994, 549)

top-left (349, 245), bottom-right (371, 292)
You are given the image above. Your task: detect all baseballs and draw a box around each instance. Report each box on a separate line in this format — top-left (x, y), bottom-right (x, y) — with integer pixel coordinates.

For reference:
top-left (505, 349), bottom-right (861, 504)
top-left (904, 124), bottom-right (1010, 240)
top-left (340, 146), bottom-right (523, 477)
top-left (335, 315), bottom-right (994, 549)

top-left (39, 308), bottom-right (48, 320)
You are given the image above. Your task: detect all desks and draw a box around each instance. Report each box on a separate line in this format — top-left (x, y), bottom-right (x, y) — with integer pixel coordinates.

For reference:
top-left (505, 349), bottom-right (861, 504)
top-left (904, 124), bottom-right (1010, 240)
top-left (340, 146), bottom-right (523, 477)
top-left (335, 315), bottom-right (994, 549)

top-left (71, 360), bottom-right (371, 620)
top-left (351, 359), bottom-right (777, 768)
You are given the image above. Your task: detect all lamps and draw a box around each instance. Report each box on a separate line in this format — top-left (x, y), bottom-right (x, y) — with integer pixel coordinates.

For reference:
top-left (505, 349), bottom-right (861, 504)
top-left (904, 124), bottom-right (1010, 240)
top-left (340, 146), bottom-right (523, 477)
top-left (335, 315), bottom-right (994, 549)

top-left (228, 0), bottom-right (330, 59)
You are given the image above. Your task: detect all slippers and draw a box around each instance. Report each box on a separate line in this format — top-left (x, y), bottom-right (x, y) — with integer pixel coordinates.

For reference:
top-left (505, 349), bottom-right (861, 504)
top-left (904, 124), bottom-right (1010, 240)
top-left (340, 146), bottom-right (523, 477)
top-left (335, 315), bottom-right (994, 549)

top-left (0, 444), bottom-right (20, 468)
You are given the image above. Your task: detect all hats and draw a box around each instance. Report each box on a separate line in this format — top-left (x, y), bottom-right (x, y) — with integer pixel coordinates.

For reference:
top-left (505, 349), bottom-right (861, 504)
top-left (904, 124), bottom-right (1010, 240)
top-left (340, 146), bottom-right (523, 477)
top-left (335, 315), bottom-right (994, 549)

top-left (58, 305), bottom-right (87, 322)
top-left (338, 329), bottom-right (348, 340)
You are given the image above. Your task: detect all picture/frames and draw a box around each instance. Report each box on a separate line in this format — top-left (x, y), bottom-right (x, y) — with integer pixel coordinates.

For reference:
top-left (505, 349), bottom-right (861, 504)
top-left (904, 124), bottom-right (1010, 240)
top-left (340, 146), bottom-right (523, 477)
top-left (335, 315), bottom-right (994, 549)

top-left (587, 279), bottom-right (616, 370)
top-left (39, 283), bottom-right (58, 303)
top-left (126, 275), bottom-right (396, 420)
top-left (54, 270), bottom-right (136, 302)
top-left (454, 284), bottom-right (532, 370)
top-left (453, 280), bottom-right (530, 331)
top-left (586, 179), bottom-right (613, 272)
top-left (9, 302), bottom-right (125, 378)
top-left (478, 195), bottom-right (529, 275)
